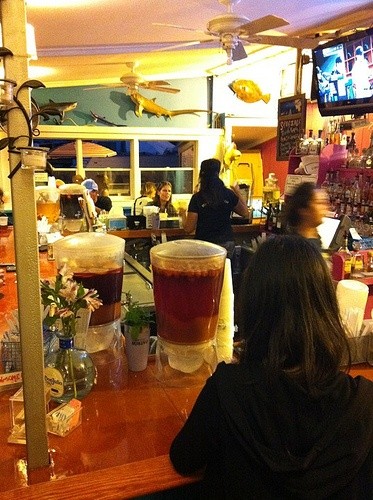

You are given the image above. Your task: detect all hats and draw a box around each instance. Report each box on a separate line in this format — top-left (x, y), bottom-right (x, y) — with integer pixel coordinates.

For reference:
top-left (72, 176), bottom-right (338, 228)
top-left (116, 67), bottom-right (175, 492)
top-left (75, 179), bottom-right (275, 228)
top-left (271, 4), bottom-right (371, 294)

top-left (201, 159), bottom-right (220, 171)
top-left (80, 178), bottom-right (98, 192)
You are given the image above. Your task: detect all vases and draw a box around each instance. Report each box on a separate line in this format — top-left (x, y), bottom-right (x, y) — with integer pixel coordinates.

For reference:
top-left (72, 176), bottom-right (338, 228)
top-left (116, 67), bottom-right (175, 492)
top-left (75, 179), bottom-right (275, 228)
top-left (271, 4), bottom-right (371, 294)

top-left (44, 333), bottom-right (96, 404)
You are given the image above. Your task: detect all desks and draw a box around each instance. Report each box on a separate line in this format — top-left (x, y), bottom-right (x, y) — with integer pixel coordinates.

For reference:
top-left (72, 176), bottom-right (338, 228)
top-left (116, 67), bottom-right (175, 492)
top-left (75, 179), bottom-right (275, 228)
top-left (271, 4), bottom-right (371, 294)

top-left (0, 241), bottom-right (373, 500)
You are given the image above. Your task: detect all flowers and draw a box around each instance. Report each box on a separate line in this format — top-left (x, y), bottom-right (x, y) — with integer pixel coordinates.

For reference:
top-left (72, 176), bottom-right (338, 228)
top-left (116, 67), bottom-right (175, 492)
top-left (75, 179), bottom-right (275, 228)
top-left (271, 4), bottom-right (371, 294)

top-left (40, 263), bottom-right (103, 335)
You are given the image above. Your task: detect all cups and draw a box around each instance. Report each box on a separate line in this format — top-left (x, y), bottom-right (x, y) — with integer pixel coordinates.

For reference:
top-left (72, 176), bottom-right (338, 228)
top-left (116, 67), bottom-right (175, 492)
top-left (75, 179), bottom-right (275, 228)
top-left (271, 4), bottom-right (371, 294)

top-left (53, 233), bottom-right (126, 364)
top-left (59, 184), bottom-right (89, 218)
top-left (35, 187), bottom-right (59, 222)
top-left (150, 237), bottom-right (228, 387)
top-left (215, 259), bottom-right (234, 369)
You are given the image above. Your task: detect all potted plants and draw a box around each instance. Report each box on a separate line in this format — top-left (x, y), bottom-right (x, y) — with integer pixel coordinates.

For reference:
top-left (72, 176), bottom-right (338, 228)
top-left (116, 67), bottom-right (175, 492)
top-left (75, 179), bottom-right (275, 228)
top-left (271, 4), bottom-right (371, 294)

top-left (123, 301), bottom-right (151, 372)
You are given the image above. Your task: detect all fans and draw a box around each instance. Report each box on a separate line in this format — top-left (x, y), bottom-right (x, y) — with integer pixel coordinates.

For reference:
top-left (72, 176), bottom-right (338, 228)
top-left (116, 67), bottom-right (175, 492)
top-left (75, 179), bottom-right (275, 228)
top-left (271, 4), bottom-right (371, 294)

top-left (83, 60), bottom-right (181, 98)
top-left (150, 0), bottom-right (319, 65)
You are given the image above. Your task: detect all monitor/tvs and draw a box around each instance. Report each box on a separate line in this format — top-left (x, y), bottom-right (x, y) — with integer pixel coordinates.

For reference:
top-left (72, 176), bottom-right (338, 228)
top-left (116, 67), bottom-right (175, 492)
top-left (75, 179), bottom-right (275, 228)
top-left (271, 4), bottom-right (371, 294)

top-left (312, 27), bottom-right (373, 117)
top-left (316, 211), bottom-right (351, 250)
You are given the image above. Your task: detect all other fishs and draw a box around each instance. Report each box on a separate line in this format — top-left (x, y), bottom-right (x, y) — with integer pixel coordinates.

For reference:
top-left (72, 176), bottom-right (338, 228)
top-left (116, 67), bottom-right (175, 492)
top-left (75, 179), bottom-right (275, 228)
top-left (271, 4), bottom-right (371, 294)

top-left (89, 110), bottom-right (127, 127)
top-left (230, 79), bottom-right (271, 104)
top-left (38, 98), bottom-right (78, 122)
top-left (130, 91), bottom-right (216, 121)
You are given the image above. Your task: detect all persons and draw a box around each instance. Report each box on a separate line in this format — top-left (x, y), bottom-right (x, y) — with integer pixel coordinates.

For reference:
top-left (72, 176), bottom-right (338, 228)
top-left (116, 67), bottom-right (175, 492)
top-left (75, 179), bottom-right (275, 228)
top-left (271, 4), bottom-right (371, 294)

top-left (131, 182), bottom-right (157, 217)
top-left (81, 178), bottom-right (112, 217)
top-left (169, 234), bottom-right (373, 500)
top-left (147, 181), bottom-right (180, 229)
top-left (329, 57), bottom-right (343, 83)
top-left (351, 46), bottom-right (373, 98)
top-left (178, 159), bottom-right (248, 271)
top-left (284, 182), bottom-right (331, 252)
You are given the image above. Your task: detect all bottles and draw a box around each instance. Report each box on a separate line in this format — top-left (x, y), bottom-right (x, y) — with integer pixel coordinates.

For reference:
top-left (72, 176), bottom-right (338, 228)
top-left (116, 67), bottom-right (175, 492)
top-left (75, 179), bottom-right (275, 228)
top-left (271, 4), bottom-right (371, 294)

top-left (339, 231), bottom-right (351, 276)
top-left (305, 130), bottom-right (373, 224)
